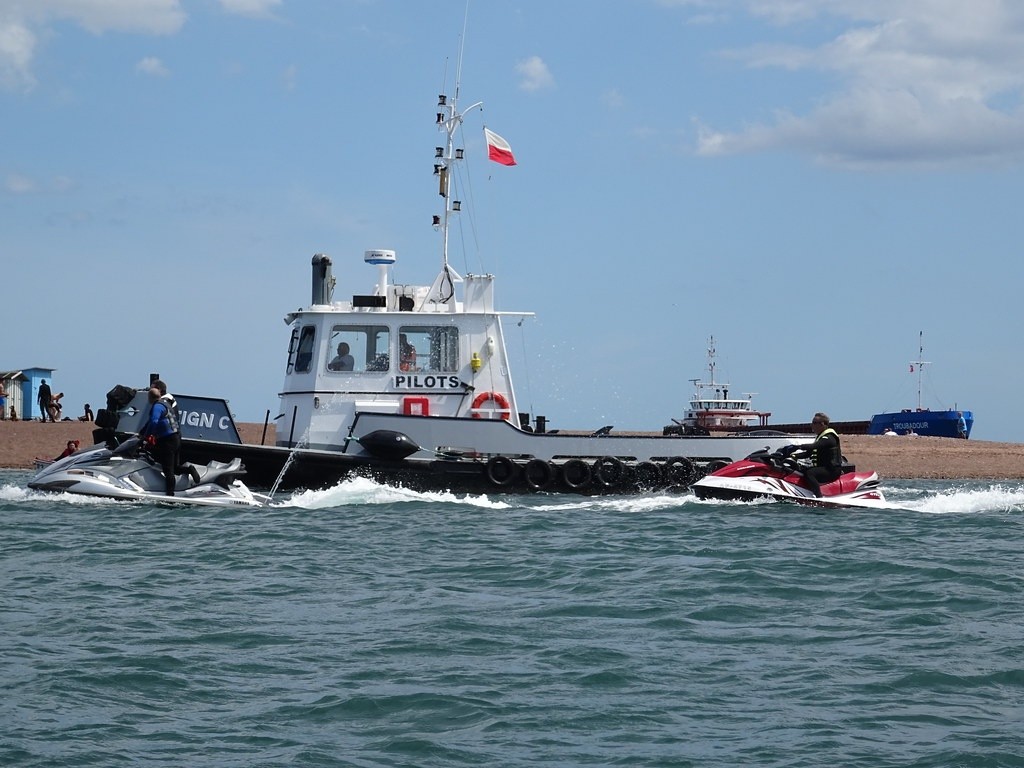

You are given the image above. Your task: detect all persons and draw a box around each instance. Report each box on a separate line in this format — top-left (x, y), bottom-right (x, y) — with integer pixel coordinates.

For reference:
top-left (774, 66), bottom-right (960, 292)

top-left (0, 378), bottom-right (9, 420)
top-left (957, 412), bottom-right (967, 439)
top-left (78, 404), bottom-right (94, 422)
top-left (328, 342), bottom-right (354, 371)
top-left (786, 413), bottom-right (843, 498)
top-left (399, 333), bottom-right (416, 371)
top-left (905, 429), bottom-right (918, 435)
top-left (138, 380), bottom-right (200, 497)
top-left (52, 440), bottom-right (81, 462)
top-left (37, 379), bottom-right (64, 423)
top-left (884, 428), bottom-right (898, 435)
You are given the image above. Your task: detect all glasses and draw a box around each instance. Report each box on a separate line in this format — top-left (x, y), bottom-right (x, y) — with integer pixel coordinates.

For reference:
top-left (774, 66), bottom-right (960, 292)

top-left (812, 421), bottom-right (821, 425)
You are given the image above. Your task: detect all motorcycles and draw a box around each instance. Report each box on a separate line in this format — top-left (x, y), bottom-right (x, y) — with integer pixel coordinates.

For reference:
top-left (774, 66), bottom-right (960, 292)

top-left (687, 443), bottom-right (884, 508)
top-left (28, 434), bottom-right (263, 508)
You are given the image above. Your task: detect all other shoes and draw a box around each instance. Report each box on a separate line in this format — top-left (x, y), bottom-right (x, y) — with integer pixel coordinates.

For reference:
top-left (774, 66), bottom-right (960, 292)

top-left (52, 421), bottom-right (55, 423)
top-left (42, 420), bottom-right (46, 423)
top-left (188, 465), bottom-right (200, 483)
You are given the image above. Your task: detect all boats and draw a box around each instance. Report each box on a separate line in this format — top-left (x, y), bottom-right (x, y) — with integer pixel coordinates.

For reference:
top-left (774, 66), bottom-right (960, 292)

top-left (663, 330), bottom-right (974, 439)
top-left (92, 0), bottom-right (853, 496)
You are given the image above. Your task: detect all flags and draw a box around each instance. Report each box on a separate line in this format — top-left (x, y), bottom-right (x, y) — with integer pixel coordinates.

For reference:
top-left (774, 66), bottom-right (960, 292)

top-left (485, 128), bottom-right (517, 166)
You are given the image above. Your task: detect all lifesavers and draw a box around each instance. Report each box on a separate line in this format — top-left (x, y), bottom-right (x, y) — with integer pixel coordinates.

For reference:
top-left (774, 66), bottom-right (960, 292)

top-left (593, 456), bottom-right (625, 489)
top-left (705, 459), bottom-right (730, 475)
top-left (665, 455), bottom-right (698, 490)
top-left (524, 458), bottom-right (554, 491)
top-left (485, 455), bottom-right (516, 487)
top-left (631, 462), bottom-right (664, 493)
top-left (471, 392), bottom-right (510, 422)
top-left (562, 459), bottom-right (593, 491)
